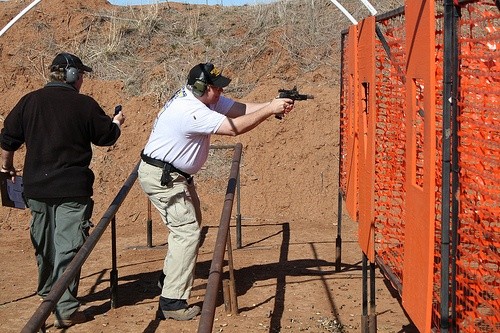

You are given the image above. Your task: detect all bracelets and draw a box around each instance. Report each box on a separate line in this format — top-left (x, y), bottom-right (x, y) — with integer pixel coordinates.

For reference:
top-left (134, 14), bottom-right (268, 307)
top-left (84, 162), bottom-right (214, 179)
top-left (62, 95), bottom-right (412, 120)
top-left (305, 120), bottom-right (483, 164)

top-left (3, 165), bottom-right (13, 171)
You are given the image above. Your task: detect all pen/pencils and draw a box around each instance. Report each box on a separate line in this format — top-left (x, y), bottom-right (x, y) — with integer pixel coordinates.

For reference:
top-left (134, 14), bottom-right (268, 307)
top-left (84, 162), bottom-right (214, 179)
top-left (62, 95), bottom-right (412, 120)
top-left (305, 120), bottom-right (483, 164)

top-left (14, 168), bottom-right (22, 171)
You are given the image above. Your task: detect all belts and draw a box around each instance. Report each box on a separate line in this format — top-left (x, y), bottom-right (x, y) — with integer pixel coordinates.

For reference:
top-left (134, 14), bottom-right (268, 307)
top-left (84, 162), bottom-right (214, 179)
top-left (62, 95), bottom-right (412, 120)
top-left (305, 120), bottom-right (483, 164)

top-left (140, 149), bottom-right (190, 179)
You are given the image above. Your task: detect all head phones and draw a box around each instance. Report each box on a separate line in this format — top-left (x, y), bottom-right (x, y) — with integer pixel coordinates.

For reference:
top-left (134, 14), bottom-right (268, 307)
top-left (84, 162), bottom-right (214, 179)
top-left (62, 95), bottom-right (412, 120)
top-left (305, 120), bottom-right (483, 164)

top-left (59, 52), bottom-right (79, 84)
top-left (192, 63), bottom-right (214, 97)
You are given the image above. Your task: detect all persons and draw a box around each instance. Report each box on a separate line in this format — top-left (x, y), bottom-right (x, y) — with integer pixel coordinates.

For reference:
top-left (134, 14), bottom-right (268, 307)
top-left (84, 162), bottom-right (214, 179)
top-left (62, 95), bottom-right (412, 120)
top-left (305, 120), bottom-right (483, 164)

top-left (0, 52), bottom-right (126, 328)
top-left (137, 63), bottom-right (294, 321)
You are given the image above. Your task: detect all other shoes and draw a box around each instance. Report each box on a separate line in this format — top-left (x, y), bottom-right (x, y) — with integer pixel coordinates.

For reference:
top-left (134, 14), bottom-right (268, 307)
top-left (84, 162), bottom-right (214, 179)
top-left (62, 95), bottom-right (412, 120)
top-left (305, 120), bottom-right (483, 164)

top-left (44, 307), bottom-right (88, 327)
top-left (156, 302), bottom-right (201, 321)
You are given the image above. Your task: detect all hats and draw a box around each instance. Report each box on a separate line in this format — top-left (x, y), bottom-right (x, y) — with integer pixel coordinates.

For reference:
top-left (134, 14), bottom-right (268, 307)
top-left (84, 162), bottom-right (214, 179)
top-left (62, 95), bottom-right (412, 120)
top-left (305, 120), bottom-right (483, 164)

top-left (187, 62), bottom-right (232, 88)
top-left (52, 53), bottom-right (93, 72)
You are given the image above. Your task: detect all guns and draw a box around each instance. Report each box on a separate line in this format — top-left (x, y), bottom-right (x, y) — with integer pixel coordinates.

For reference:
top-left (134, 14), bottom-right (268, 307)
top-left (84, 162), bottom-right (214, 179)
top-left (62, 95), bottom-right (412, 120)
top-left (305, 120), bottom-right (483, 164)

top-left (275, 85), bottom-right (314, 120)
